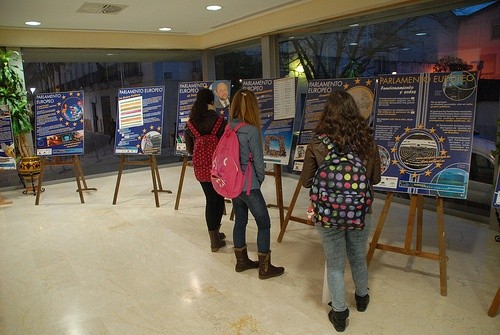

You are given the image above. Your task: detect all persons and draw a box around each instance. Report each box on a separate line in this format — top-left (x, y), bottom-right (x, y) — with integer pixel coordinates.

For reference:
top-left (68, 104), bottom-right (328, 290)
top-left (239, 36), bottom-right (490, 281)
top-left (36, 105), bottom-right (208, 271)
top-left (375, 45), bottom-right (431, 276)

top-left (301, 90), bottom-right (381, 332)
top-left (184, 89), bottom-right (227, 252)
top-left (107, 117), bottom-right (118, 146)
top-left (227, 89), bottom-right (285, 279)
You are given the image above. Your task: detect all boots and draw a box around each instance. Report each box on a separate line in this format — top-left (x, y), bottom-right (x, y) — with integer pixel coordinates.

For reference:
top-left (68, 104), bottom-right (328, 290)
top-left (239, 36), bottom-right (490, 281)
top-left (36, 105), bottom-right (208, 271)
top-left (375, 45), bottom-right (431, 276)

top-left (354, 291), bottom-right (370, 313)
top-left (328, 302), bottom-right (351, 332)
top-left (256, 250), bottom-right (285, 279)
top-left (206, 230), bottom-right (226, 252)
top-left (231, 244), bottom-right (258, 274)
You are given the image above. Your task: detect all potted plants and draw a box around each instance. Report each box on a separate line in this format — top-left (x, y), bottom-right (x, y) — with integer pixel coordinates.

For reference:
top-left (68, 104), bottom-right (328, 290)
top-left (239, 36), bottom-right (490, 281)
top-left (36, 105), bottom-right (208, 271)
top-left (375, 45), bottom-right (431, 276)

top-left (0, 48), bottom-right (45, 196)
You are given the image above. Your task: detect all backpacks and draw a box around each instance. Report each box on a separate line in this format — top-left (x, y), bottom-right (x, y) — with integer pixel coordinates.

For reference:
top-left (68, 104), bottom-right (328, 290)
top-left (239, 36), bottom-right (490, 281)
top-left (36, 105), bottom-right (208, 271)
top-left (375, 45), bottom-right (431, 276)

top-left (210, 123), bottom-right (256, 203)
top-left (185, 113), bottom-right (224, 185)
top-left (306, 132), bottom-right (373, 233)
